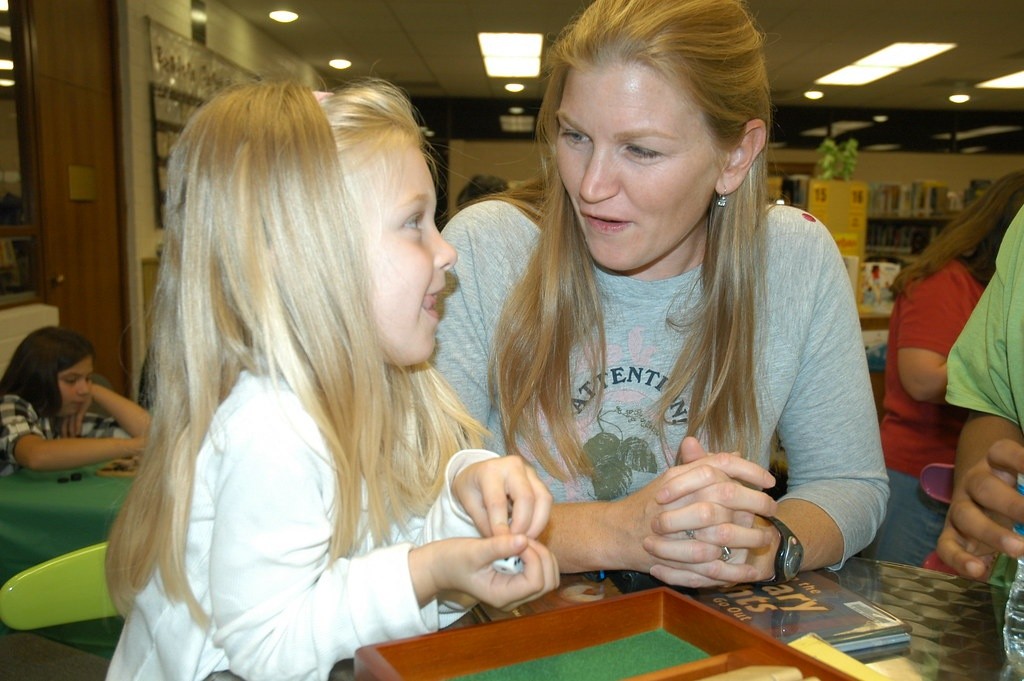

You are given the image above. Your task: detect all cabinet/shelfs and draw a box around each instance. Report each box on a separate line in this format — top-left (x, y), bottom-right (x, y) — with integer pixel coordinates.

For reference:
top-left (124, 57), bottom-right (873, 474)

top-left (866, 204), bottom-right (970, 270)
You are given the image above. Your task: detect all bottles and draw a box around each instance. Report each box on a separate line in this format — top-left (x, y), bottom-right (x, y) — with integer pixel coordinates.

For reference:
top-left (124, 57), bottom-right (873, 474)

top-left (1002, 472), bottom-right (1024, 681)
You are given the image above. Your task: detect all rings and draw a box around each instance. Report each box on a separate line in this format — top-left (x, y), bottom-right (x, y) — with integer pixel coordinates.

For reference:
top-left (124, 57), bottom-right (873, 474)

top-left (720, 547), bottom-right (731, 561)
top-left (685, 530), bottom-right (694, 539)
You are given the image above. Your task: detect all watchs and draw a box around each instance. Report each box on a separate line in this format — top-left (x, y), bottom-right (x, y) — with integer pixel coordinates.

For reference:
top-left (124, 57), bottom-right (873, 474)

top-left (760, 590), bottom-right (799, 638)
top-left (755, 513), bottom-right (804, 585)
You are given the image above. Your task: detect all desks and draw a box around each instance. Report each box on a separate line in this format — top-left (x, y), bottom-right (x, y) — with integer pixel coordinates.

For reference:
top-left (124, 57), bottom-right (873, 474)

top-left (533, 567), bottom-right (1005, 681)
top-left (0, 455), bottom-right (151, 655)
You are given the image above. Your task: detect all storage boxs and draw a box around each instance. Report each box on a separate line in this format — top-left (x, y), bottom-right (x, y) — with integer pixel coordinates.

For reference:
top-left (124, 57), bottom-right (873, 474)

top-left (353, 588), bottom-right (859, 679)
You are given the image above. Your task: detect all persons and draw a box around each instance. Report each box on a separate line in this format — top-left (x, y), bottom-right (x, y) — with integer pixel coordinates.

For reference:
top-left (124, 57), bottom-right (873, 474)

top-left (0, 191), bottom-right (31, 258)
top-left (923, 199), bottom-right (1024, 579)
top-left (428, 0), bottom-right (889, 587)
top-left (103, 72), bottom-right (561, 681)
top-left (0, 323), bottom-right (153, 477)
top-left (875, 169), bottom-right (1024, 568)
